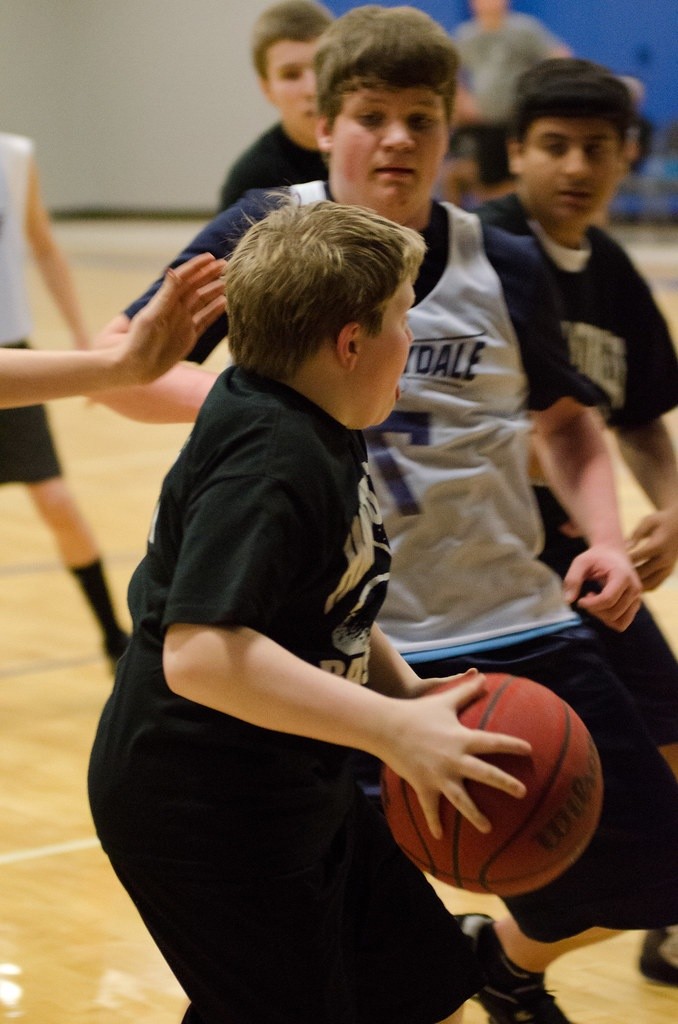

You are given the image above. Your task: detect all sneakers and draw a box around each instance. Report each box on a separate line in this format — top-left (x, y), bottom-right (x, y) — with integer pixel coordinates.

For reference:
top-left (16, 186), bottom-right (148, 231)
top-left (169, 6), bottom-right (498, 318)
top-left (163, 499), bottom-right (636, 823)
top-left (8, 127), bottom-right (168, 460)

top-left (639, 926), bottom-right (678, 987)
top-left (453, 913), bottom-right (571, 1024)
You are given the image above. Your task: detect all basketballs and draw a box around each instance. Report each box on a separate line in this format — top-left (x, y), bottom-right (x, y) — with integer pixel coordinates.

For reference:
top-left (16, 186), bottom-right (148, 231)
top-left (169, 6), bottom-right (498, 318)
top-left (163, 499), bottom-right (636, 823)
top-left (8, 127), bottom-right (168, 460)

top-left (380, 670), bottom-right (605, 897)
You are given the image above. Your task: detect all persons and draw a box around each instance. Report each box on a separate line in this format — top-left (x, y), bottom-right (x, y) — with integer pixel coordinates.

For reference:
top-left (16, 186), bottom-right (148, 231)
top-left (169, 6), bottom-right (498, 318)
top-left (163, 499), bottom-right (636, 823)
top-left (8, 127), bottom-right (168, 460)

top-left (82, 0), bottom-right (678, 1024)
top-left (468, 56), bottom-right (678, 986)
top-left (211, 0), bottom-right (337, 212)
top-left (88, 192), bottom-right (532, 1024)
top-left (0, 251), bottom-right (227, 411)
top-left (0, 130), bottom-right (126, 666)
top-left (435, 0), bottom-right (646, 202)
